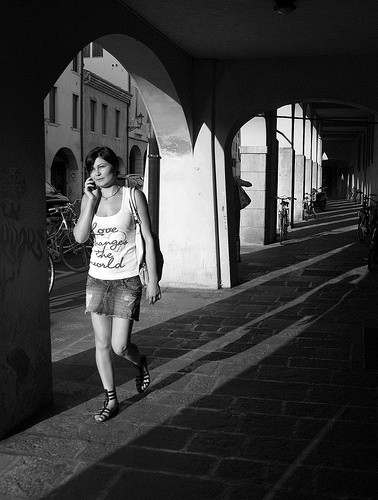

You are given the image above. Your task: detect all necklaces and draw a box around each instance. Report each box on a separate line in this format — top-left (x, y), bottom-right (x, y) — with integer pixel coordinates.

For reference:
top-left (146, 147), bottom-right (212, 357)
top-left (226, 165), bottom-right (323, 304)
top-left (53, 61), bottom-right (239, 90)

top-left (101, 185), bottom-right (122, 200)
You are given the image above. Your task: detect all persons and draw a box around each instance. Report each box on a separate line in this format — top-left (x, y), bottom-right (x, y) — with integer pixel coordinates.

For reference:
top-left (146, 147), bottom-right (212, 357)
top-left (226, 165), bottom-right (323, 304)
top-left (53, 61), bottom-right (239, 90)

top-left (72, 146), bottom-right (162, 421)
top-left (233, 175), bottom-right (252, 263)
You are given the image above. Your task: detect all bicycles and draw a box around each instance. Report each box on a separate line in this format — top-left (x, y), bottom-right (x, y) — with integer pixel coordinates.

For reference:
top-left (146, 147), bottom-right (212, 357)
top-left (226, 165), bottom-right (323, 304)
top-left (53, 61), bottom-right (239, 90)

top-left (277, 196), bottom-right (297, 246)
top-left (45, 199), bottom-right (95, 295)
top-left (302, 186), bottom-right (327, 222)
top-left (346, 187), bottom-right (362, 202)
top-left (358, 193), bottom-right (378, 245)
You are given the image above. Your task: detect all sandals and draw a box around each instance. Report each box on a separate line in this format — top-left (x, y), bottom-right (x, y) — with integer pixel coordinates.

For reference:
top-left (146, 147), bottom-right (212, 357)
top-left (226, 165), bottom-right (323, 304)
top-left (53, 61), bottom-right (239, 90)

top-left (94, 387), bottom-right (119, 422)
top-left (132, 356), bottom-right (150, 394)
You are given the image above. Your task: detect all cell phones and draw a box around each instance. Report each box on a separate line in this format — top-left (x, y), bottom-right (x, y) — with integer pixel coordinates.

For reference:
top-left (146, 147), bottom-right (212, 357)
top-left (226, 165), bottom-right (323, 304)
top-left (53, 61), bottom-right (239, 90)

top-left (89, 179), bottom-right (97, 190)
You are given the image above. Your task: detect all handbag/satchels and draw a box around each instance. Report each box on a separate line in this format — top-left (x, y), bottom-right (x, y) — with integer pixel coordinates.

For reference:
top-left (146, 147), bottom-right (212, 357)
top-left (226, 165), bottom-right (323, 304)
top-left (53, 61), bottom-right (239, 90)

top-left (235, 176), bottom-right (251, 210)
top-left (128, 187), bottom-right (164, 286)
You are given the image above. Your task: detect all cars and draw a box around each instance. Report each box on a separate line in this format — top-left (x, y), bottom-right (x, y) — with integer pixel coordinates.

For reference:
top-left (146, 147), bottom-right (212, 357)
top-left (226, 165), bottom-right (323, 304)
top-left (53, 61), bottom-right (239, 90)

top-left (45, 181), bottom-right (70, 215)
